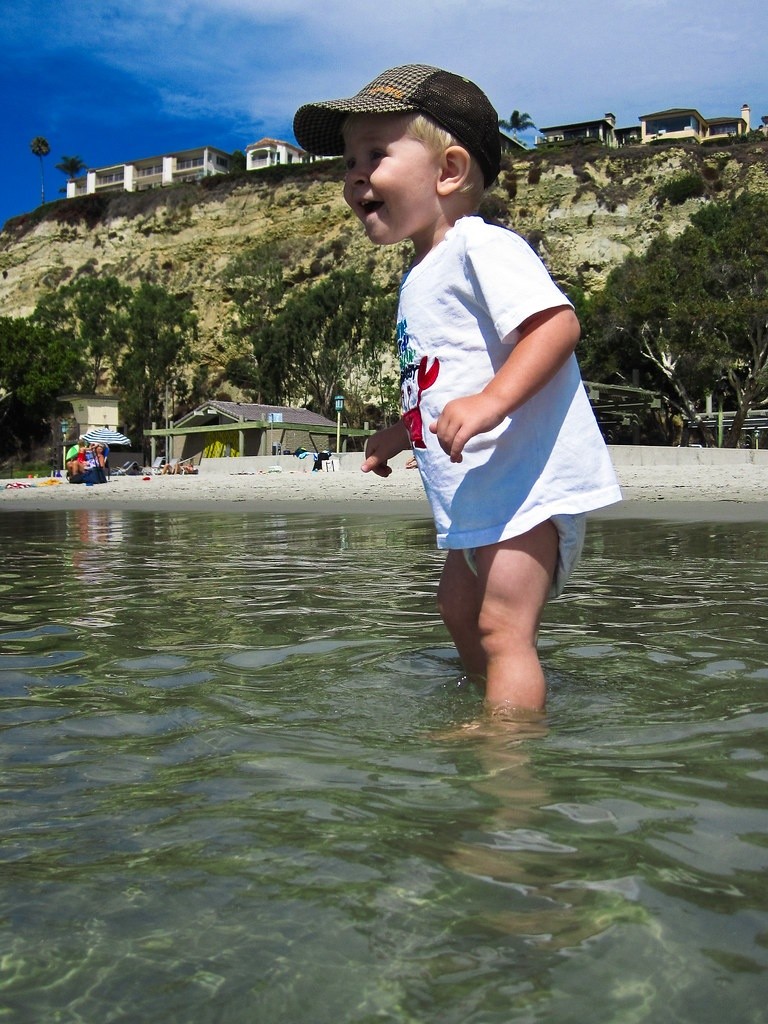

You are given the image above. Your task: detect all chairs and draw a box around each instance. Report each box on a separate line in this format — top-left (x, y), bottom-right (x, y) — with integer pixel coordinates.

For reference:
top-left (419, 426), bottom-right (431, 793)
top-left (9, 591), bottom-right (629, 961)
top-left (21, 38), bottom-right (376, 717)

top-left (87, 444), bottom-right (109, 469)
top-left (111, 457), bottom-right (197, 475)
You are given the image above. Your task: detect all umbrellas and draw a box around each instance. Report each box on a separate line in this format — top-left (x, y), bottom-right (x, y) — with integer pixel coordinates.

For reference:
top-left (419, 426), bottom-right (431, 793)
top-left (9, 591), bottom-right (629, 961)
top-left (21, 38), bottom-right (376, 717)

top-left (65, 443), bottom-right (80, 460)
top-left (81, 424), bottom-right (131, 480)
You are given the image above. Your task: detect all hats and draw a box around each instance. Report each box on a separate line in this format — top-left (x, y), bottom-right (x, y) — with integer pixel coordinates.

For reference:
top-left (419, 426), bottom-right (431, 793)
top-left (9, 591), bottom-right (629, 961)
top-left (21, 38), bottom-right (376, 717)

top-left (293, 65), bottom-right (504, 190)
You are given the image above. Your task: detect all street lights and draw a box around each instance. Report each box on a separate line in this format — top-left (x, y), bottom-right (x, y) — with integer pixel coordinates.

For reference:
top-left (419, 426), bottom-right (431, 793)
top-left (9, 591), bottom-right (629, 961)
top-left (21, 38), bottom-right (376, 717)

top-left (334, 395), bottom-right (346, 453)
top-left (60, 418), bottom-right (69, 470)
top-left (164, 378), bottom-right (177, 476)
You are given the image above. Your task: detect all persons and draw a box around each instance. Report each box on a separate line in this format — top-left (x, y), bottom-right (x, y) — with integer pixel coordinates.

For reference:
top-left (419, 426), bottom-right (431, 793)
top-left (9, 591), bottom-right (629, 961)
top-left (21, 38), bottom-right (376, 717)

top-left (406, 457), bottom-right (418, 469)
top-left (161, 463), bottom-right (193, 476)
top-left (294, 63), bottom-right (622, 718)
top-left (65, 441), bottom-right (110, 480)
